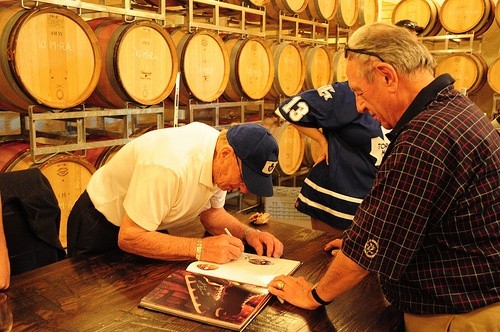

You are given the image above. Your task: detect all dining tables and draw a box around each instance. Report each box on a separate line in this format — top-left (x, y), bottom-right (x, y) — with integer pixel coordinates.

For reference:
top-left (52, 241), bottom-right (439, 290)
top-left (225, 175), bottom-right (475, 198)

top-left (0, 208), bottom-right (389, 332)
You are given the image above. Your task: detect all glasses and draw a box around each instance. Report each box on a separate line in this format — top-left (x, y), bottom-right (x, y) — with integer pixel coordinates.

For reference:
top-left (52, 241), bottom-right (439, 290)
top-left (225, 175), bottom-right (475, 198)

top-left (344, 43), bottom-right (385, 62)
top-left (235, 155), bottom-right (245, 185)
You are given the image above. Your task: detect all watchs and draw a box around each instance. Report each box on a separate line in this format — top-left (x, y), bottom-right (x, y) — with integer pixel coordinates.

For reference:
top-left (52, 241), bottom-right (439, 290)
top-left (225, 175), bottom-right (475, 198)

top-left (311, 282), bottom-right (335, 306)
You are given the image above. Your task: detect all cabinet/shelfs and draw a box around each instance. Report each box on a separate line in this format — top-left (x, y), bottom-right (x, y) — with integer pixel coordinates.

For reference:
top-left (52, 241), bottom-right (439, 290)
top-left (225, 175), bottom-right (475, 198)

top-left (0, 0), bottom-right (500, 213)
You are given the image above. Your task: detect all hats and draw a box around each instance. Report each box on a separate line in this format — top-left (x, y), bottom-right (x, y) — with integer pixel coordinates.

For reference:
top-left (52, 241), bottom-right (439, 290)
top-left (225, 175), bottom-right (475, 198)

top-left (395, 20), bottom-right (424, 35)
top-left (226, 122), bottom-right (279, 197)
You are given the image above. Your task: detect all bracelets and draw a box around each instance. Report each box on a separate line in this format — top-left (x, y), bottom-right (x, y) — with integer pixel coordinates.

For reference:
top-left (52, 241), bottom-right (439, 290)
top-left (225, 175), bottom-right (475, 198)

top-left (195, 238), bottom-right (203, 261)
top-left (243, 227), bottom-right (261, 240)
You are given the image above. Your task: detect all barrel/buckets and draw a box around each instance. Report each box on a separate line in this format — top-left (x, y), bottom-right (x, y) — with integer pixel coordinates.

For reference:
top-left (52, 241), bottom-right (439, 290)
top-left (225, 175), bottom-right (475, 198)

top-left (391, 0), bottom-right (500, 96)
top-left (0, 123), bottom-right (179, 249)
top-left (76, 16), bottom-right (179, 109)
top-left (217, 33), bottom-right (274, 105)
top-left (265, 42), bottom-right (348, 102)
top-left (162, 26), bottom-right (230, 105)
top-left (133, 0), bottom-right (382, 38)
top-left (198, 114), bottom-right (326, 177)
top-left (0, 4), bottom-right (102, 114)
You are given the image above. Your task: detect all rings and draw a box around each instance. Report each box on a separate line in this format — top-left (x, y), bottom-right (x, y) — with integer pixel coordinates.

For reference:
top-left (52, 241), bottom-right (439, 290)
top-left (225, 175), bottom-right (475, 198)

top-left (277, 281), bottom-right (284, 289)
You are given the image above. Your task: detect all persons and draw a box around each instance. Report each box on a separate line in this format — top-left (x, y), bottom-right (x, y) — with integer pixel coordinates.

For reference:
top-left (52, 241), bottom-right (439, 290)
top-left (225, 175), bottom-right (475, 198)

top-left (274, 81), bottom-right (393, 236)
top-left (266, 22), bottom-right (500, 332)
top-left (66, 122), bottom-right (284, 265)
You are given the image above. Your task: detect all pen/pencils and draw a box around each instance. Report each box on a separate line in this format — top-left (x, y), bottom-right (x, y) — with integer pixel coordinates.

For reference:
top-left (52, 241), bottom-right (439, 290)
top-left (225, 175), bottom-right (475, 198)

top-left (224, 228), bottom-right (245, 257)
